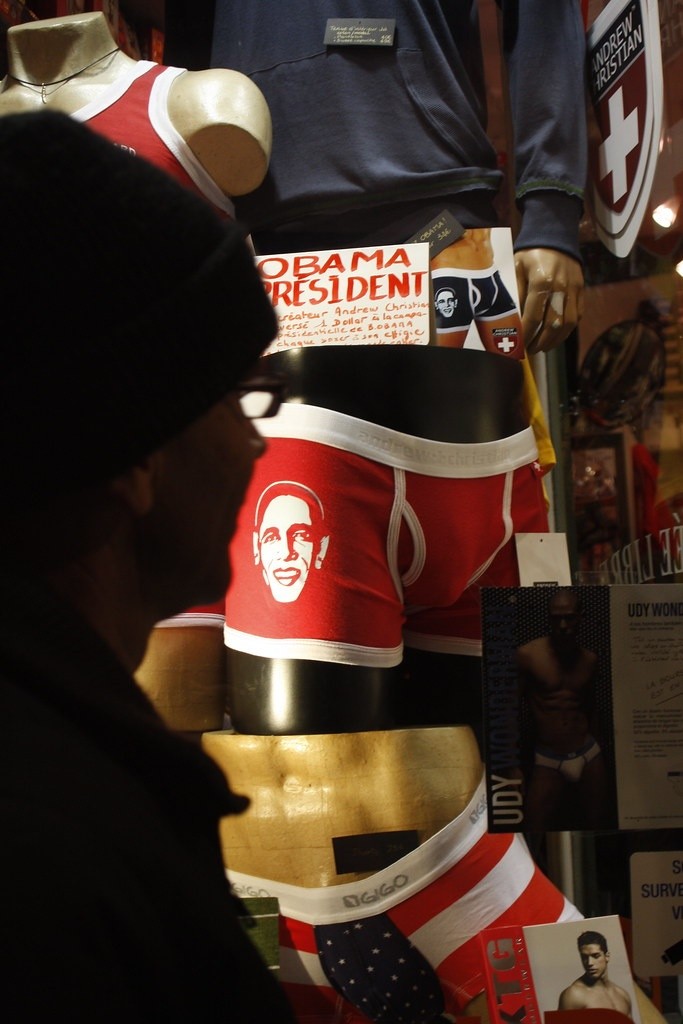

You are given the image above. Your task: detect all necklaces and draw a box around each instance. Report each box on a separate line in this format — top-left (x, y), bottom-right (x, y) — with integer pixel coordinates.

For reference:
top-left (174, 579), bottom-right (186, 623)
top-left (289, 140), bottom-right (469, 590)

top-left (8, 47), bottom-right (120, 105)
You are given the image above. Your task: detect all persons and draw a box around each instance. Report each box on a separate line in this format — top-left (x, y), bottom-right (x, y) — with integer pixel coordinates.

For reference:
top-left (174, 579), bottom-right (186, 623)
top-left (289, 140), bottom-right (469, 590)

top-left (0, 0), bottom-right (672, 1024)
top-left (558, 931), bottom-right (632, 1018)
top-left (0, 109), bottom-right (304, 1024)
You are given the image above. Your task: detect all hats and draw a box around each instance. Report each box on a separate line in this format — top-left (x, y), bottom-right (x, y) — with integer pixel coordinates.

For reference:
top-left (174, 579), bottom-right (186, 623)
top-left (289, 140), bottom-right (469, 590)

top-left (1, 108), bottom-right (278, 538)
